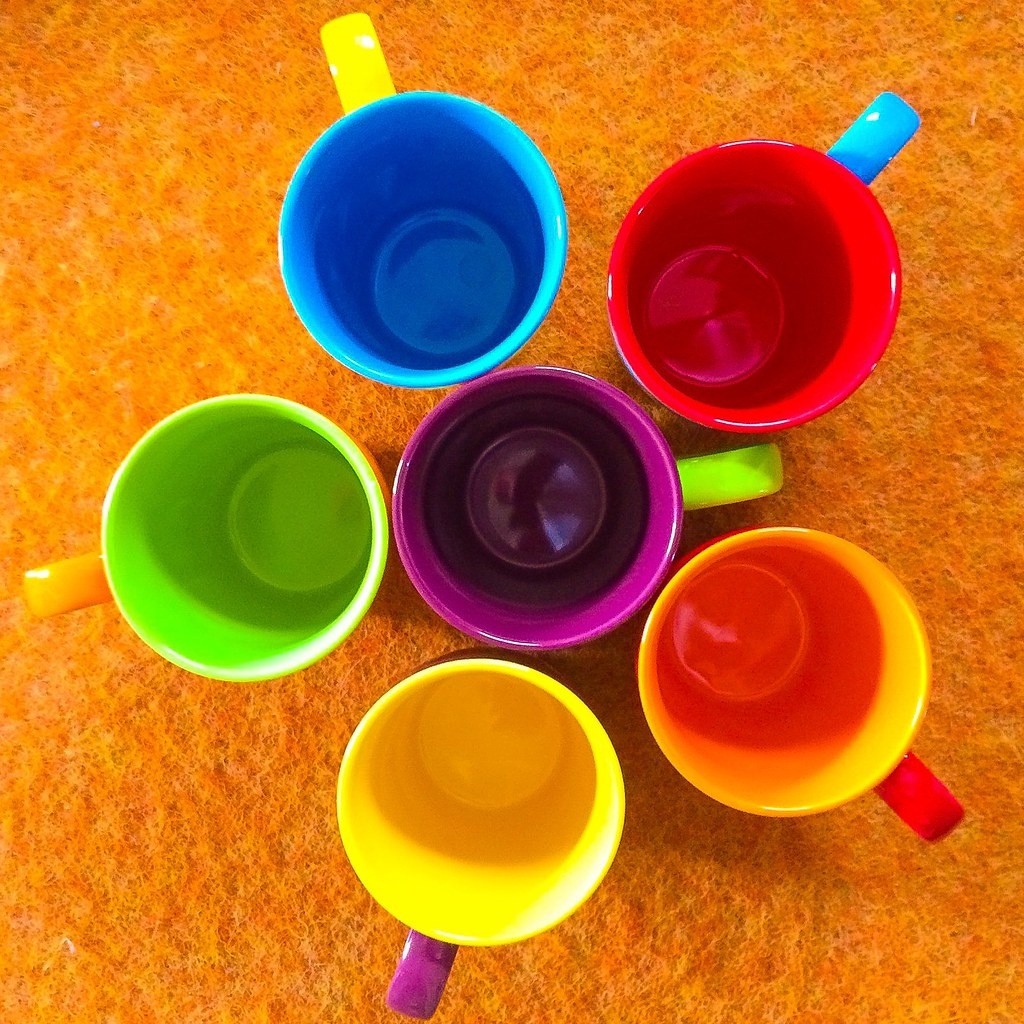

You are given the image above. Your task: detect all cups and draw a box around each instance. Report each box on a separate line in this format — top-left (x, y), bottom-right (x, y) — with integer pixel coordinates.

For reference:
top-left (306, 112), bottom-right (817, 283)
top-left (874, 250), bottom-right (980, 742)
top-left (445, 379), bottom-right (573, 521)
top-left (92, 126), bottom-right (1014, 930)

top-left (24, 393), bottom-right (388, 681)
top-left (393, 365), bottom-right (782, 652)
top-left (638, 527), bottom-right (963, 845)
top-left (607, 93), bottom-right (923, 432)
top-left (336, 658), bottom-right (626, 1020)
top-left (279, 12), bottom-right (569, 386)
